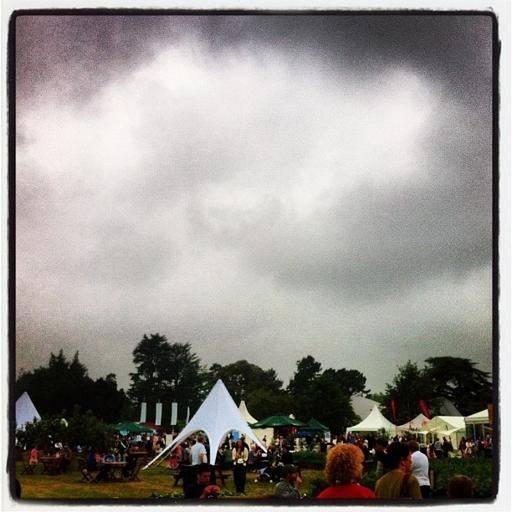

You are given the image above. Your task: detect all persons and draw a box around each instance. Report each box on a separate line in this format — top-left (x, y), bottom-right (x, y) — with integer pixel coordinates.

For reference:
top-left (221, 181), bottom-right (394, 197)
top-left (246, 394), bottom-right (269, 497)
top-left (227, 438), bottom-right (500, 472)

top-left (17, 413), bottom-right (493, 500)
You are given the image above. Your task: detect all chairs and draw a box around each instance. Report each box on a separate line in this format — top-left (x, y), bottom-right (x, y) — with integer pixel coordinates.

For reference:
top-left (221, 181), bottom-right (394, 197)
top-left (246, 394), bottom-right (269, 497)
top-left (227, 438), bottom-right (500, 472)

top-left (20, 440), bottom-right (271, 494)
